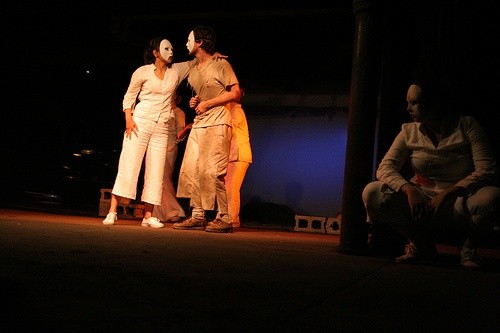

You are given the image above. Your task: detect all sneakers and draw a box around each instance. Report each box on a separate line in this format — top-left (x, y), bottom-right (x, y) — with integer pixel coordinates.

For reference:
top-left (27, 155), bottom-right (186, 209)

top-left (102, 212), bottom-right (117, 225)
top-left (141, 217), bottom-right (164, 228)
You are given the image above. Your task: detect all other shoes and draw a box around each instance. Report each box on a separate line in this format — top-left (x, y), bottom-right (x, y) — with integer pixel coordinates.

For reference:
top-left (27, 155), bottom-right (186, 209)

top-left (232, 223), bottom-right (240, 229)
top-left (459, 248), bottom-right (481, 270)
top-left (395, 245), bottom-right (430, 264)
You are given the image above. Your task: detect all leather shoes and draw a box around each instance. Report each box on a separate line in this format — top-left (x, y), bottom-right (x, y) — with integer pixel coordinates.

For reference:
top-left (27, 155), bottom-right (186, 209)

top-left (205, 218), bottom-right (233, 233)
top-left (173, 217), bottom-right (206, 229)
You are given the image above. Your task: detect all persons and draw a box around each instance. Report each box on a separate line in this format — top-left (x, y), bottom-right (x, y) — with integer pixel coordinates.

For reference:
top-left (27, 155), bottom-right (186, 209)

top-left (208, 90), bottom-right (252, 228)
top-left (172, 28), bottom-right (241, 232)
top-left (102, 36), bottom-right (229, 228)
top-left (362, 74), bottom-right (500, 268)
top-left (153, 91), bottom-right (186, 223)
